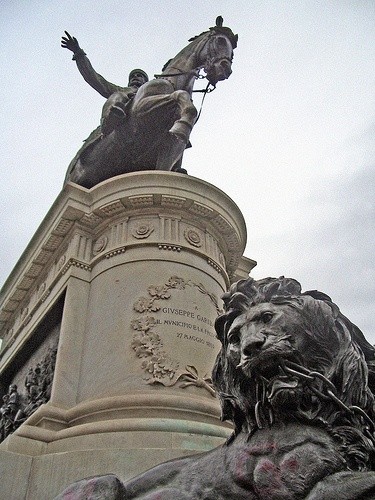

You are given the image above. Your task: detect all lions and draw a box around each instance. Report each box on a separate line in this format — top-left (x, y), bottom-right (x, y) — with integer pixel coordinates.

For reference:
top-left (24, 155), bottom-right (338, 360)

top-left (51, 275), bottom-right (375, 500)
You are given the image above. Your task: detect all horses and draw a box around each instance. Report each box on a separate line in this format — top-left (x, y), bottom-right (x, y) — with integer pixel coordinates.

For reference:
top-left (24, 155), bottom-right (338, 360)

top-left (65, 15), bottom-right (238, 191)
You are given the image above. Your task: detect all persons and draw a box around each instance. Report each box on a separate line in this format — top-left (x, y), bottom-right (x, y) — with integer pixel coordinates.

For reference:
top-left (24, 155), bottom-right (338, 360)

top-left (60, 30), bottom-right (191, 176)
